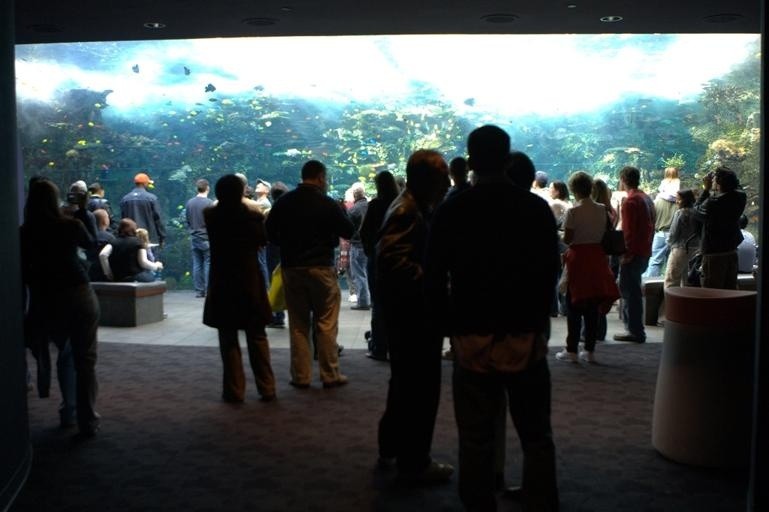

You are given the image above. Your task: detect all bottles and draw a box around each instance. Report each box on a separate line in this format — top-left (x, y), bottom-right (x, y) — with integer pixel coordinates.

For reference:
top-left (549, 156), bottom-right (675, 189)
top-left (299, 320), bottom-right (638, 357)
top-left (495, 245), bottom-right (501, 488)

top-left (154, 267), bottom-right (162, 281)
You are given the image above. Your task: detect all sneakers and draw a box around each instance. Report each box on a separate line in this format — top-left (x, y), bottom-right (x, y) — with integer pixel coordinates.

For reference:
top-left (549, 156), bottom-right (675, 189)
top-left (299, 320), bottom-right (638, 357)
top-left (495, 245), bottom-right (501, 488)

top-left (505, 485), bottom-right (523, 498)
top-left (614, 333), bottom-right (645, 343)
top-left (555, 348), bottom-right (578, 362)
top-left (579, 351), bottom-right (594, 361)
top-left (424, 459), bottom-right (455, 478)
top-left (324, 375), bottom-right (348, 388)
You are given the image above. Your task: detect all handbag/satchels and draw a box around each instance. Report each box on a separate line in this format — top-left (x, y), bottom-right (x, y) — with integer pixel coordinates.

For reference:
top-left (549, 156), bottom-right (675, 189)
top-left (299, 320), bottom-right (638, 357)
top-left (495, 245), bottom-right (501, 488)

top-left (604, 206), bottom-right (626, 256)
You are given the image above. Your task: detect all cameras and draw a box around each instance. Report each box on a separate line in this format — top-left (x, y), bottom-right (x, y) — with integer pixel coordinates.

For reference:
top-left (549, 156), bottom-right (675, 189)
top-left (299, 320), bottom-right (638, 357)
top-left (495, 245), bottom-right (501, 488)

top-left (67, 193), bottom-right (84, 204)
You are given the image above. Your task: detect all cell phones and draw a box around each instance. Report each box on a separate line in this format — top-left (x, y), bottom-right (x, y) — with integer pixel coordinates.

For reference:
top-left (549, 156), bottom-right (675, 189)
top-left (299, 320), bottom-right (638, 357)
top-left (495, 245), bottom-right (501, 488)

top-left (710, 171), bottom-right (716, 178)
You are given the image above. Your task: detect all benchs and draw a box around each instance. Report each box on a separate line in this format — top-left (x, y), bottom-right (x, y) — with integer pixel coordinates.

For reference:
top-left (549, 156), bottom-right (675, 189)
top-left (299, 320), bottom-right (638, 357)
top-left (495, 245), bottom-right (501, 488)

top-left (617, 278), bottom-right (759, 326)
top-left (90, 280), bottom-right (167, 327)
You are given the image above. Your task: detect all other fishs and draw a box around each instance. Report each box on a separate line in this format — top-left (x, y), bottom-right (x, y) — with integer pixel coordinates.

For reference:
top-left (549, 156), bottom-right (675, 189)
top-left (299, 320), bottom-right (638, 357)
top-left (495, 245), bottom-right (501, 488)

top-left (184, 271), bottom-right (190, 279)
top-left (254, 85), bottom-right (264, 92)
top-left (40, 97), bottom-right (380, 230)
top-left (624, 146), bottom-right (644, 156)
top-left (132, 64), bottom-right (139, 73)
top-left (526, 143), bottom-right (533, 152)
top-left (183, 67), bottom-right (191, 76)
top-left (204, 84), bottom-right (216, 92)
top-left (465, 97), bottom-right (475, 106)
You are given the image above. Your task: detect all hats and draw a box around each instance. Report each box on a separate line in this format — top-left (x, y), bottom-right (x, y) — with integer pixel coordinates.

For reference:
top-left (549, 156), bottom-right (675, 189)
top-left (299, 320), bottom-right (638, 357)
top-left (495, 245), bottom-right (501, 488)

top-left (256, 179), bottom-right (272, 190)
top-left (134, 173), bottom-right (154, 185)
top-left (70, 180), bottom-right (87, 198)
